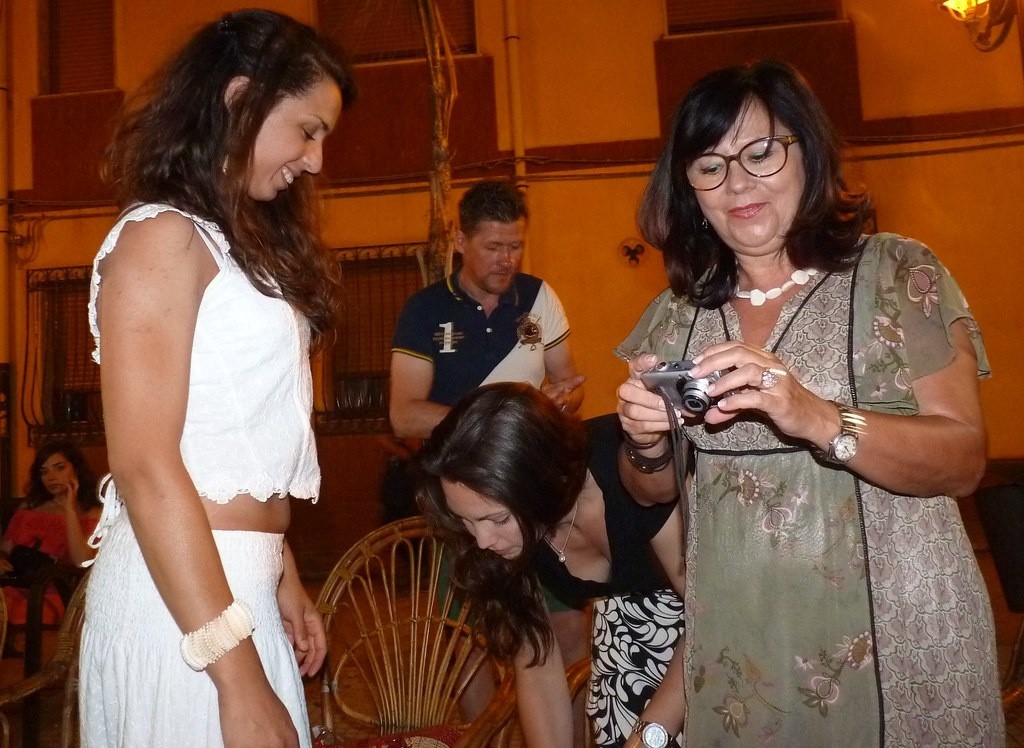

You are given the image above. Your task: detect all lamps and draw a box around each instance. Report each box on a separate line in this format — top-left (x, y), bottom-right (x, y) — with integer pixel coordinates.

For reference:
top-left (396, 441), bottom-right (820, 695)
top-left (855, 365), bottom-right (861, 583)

top-left (937, 0), bottom-right (1017, 53)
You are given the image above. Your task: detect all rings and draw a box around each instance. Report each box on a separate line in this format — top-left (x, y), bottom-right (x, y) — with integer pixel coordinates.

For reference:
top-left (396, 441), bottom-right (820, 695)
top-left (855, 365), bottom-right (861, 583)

top-left (758, 367), bottom-right (780, 390)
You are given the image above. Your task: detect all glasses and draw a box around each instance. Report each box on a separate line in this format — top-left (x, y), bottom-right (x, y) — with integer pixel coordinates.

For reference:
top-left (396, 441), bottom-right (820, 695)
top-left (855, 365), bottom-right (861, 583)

top-left (675, 129), bottom-right (818, 193)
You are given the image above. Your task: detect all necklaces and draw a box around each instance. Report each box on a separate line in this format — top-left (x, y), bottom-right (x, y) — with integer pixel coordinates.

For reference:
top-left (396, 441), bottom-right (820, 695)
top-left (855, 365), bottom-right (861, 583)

top-left (544, 498), bottom-right (578, 563)
top-left (727, 256), bottom-right (819, 307)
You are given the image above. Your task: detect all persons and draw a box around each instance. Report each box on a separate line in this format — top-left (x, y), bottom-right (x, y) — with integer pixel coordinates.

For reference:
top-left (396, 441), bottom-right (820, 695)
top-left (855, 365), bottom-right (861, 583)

top-left (77, 8), bottom-right (355, 748)
top-left (406, 377), bottom-right (686, 748)
top-left (1, 442), bottom-right (102, 625)
top-left (617, 58), bottom-right (1005, 748)
top-left (389, 173), bottom-right (587, 438)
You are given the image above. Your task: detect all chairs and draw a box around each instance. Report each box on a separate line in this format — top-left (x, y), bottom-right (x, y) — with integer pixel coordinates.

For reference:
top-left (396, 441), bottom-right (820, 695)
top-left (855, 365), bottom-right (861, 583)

top-left (0, 569), bottom-right (91, 748)
top-left (315, 515), bottom-right (517, 748)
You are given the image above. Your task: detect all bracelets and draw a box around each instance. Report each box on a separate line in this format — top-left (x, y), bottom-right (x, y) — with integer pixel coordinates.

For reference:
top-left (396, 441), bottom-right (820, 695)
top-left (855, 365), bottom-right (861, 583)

top-left (179, 598), bottom-right (256, 672)
top-left (624, 432), bottom-right (673, 474)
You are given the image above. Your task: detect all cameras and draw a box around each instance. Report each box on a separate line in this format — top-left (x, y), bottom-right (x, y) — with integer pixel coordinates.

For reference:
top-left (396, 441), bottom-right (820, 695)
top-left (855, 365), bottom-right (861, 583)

top-left (642, 360), bottom-right (742, 413)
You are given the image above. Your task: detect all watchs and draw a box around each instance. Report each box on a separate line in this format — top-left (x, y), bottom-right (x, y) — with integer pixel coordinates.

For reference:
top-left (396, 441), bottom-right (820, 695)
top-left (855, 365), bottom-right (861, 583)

top-left (631, 718), bottom-right (681, 748)
top-left (812, 399), bottom-right (858, 463)
